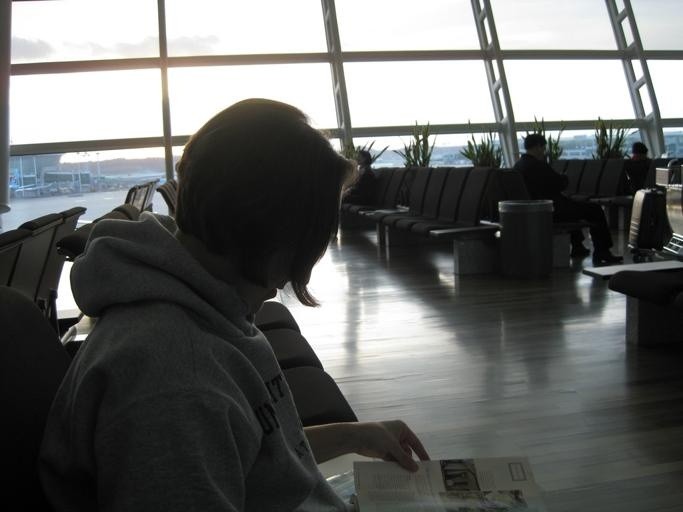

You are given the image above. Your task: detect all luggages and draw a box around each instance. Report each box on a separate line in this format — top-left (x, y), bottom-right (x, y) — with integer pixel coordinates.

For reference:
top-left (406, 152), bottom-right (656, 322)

top-left (628, 188), bottom-right (665, 254)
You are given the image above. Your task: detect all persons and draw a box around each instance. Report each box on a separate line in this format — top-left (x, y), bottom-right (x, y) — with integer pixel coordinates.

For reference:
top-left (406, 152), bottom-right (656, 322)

top-left (514, 133), bottom-right (624, 266)
top-left (340, 150), bottom-right (377, 206)
top-left (41, 97), bottom-right (431, 511)
top-left (631, 142), bottom-right (651, 160)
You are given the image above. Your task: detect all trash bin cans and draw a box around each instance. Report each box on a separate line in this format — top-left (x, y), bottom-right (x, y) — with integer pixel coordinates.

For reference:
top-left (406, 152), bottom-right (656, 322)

top-left (497, 199), bottom-right (554, 280)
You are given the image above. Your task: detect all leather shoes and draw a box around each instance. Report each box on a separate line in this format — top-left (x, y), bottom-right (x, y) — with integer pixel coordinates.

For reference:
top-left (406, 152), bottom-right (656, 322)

top-left (570, 237), bottom-right (624, 264)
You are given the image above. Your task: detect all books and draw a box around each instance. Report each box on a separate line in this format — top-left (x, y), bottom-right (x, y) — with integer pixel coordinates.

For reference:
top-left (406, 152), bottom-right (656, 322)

top-left (348, 453), bottom-right (545, 511)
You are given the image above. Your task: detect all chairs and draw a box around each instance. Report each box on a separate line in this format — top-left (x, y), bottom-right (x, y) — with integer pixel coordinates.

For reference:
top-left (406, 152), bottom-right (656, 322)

top-left (337, 154), bottom-right (626, 277)
top-left (1, 285), bottom-right (71, 510)
top-left (1, 205), bottom-right (88, 344)
top-left (124, 174), bottom-right (176, 217)
top-left (252, 301), bottom-right (361, 424)
top-left (578, 255), bottom-right (682, 347)
top-left (54, 202), bottom-right (141, 266)
